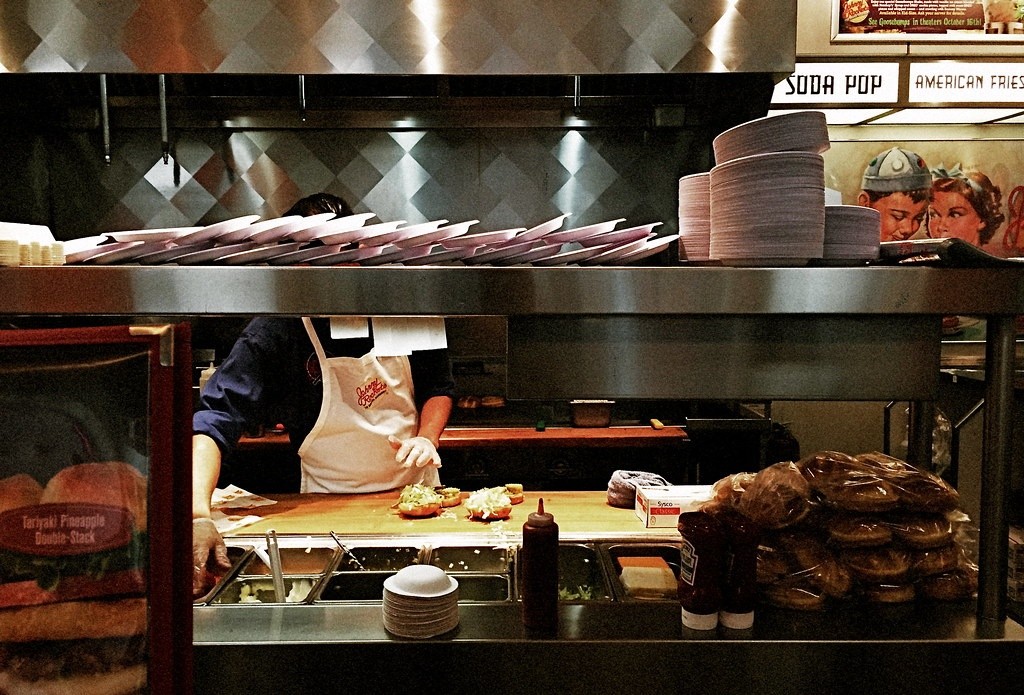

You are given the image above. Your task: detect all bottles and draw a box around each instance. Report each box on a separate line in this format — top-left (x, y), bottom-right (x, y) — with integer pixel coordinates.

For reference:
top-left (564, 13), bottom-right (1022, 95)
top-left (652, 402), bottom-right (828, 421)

top-left (676, 511), bottom-right (724, 631)
top-left (715, 507), bottom-right (757, 629)
top-left (521, 497), bottom-right (559, 642)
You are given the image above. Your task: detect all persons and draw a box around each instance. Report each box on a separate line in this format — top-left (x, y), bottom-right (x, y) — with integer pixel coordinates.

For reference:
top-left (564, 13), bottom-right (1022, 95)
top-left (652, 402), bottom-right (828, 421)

top-left (193, 192), bottom-right (457, 595)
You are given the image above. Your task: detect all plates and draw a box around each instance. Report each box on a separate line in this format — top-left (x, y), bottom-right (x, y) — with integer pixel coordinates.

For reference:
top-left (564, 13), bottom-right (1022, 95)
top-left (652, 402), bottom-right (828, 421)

top-left (60, 111), bottom-right (882, 266)
top-left (382, 564), bottom-right (460, 640)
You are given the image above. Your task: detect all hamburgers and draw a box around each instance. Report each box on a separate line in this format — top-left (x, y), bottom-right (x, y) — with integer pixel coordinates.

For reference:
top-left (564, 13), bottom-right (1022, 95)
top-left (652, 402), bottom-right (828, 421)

top-left (0, 460), bottom-right (151, 695)
top-left (465, 484), bottom-right (524, 520)
top-left (394, 479), bottom-right (461, 517)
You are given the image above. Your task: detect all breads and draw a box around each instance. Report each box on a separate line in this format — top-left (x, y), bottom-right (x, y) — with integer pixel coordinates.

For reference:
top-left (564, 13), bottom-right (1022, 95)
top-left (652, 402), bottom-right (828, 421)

top-left (457, 395), bottom-right (505, 408)
top-left (696, 452), bottom-right (980, 610)
